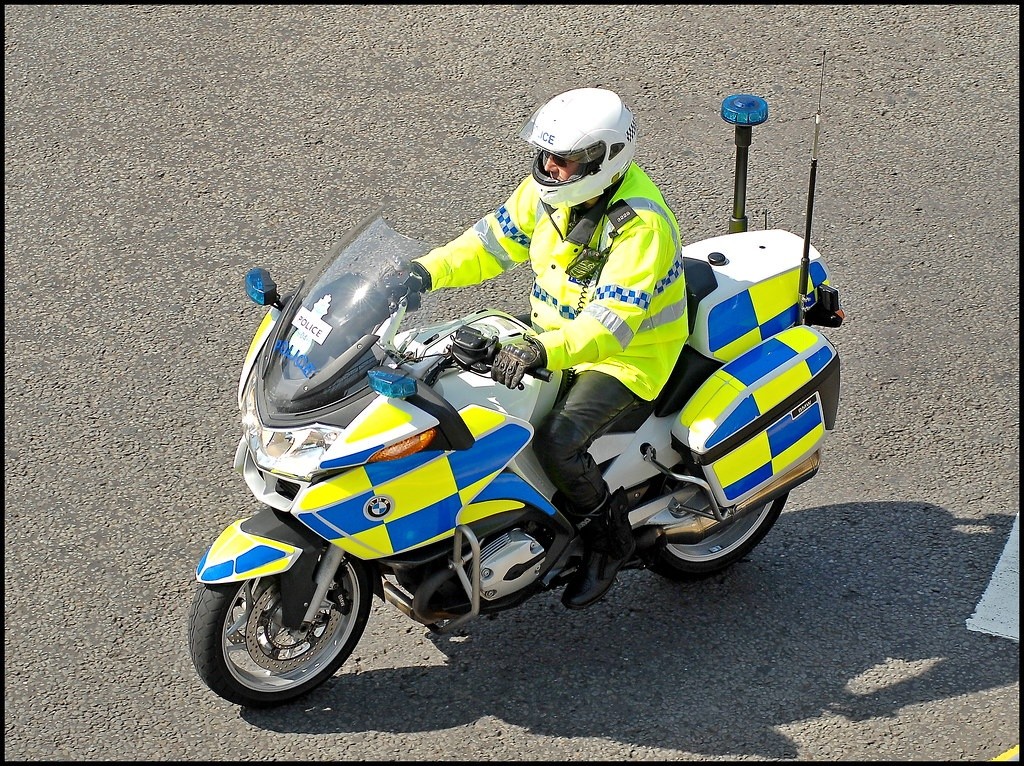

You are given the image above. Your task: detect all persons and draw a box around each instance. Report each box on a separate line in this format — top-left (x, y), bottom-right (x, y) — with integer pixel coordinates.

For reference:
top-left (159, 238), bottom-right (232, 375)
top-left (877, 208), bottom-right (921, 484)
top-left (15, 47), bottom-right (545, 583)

top-left (377, 87), bottom-right (690, 612)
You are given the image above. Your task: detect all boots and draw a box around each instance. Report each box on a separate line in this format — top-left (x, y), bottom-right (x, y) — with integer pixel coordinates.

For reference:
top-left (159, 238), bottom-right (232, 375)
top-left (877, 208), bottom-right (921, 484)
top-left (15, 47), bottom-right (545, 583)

top-left (560, 487), bottom-right (636, 610)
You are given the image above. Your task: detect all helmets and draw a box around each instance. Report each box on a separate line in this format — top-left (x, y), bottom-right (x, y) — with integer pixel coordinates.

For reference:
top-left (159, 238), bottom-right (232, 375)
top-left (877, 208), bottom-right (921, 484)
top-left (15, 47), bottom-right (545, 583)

top-left (529, 89), bottom-right (636, 209)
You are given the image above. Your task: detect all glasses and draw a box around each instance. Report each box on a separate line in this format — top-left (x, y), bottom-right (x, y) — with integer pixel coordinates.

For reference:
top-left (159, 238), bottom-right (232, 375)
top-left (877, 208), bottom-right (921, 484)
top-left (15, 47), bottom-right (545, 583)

top-left (540, 148), bottom-right (597, 168)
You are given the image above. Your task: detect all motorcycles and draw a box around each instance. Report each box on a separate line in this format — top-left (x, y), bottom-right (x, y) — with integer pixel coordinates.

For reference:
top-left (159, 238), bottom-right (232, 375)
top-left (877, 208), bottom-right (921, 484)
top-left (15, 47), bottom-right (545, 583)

top-left (186, 224), bottom-right (846, 713)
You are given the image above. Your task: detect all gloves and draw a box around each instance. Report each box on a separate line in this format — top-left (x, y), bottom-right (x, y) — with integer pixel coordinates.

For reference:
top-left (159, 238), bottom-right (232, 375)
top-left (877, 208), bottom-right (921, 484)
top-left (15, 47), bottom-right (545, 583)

top-left (376, 254), bottom-right (423, 293)
top-left (491, 334), bottom-right (547, 390)
